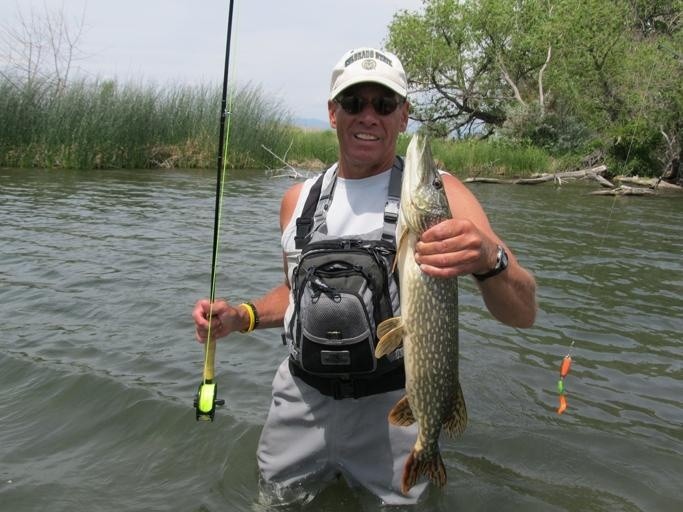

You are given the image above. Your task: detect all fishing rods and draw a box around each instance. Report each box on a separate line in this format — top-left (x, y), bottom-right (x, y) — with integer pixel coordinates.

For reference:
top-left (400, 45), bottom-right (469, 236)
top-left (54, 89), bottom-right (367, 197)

top-left (193, 1), bottom-right (234, 422)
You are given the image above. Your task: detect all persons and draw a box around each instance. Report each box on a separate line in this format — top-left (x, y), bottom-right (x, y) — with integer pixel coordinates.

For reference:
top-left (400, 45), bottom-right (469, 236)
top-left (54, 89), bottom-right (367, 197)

top-left (192, 45), bottom-right (540, 511)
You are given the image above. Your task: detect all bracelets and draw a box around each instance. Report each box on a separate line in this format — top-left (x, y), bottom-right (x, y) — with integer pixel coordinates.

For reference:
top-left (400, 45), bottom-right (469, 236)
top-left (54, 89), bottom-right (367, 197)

top-left (237, 301), bottom-right (261, 336)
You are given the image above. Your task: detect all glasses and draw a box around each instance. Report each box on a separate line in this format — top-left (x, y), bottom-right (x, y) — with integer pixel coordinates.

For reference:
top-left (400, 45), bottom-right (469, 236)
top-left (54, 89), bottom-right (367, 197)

top-left (334, 94), bottom-right (404, 116)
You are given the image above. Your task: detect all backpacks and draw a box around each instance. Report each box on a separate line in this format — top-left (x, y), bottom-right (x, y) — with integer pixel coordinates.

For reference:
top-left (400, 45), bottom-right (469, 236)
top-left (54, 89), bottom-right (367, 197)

top-left (281, 150), bottom-right (410, 403)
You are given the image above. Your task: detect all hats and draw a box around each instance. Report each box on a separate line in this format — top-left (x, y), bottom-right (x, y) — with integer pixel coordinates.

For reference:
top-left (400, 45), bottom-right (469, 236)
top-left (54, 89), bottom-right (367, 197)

top-left (328, 46), bottom-right (409, 101)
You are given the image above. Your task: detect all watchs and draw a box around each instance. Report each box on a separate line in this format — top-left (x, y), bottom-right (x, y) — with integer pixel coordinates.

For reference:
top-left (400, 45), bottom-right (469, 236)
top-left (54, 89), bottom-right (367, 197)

top-left (472, 240), bottom-right (510, 280)
